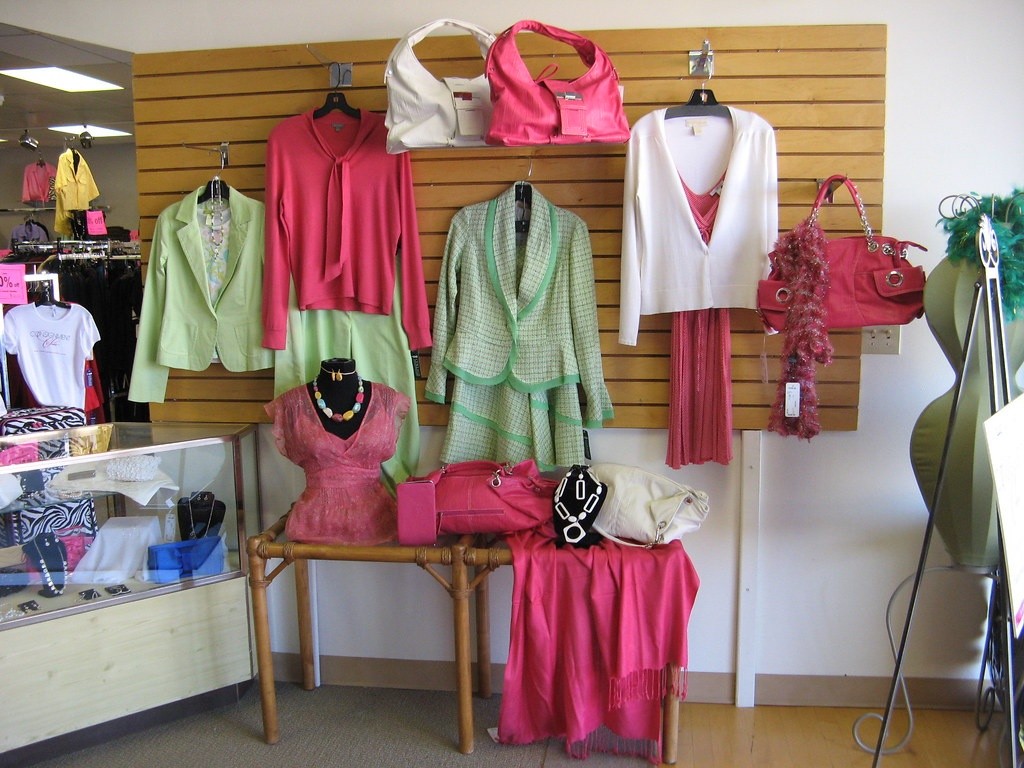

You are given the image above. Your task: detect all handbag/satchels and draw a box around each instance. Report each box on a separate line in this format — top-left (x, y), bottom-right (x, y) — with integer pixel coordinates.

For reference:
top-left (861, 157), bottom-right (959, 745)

top-left (383, 18), bottom-right (497, 154)
top-left (581, 463), bottom-right (709, 549)
top-left (484, 18), bottom-right (629, 146)
top-left (395, 460), bottom-right (560, 546)
top-left (756, 175), bottom-right (929, 332)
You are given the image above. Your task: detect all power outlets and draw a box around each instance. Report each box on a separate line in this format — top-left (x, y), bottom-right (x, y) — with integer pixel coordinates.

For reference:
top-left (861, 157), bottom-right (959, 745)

top-left (861, 324), bottom-right (901, 355)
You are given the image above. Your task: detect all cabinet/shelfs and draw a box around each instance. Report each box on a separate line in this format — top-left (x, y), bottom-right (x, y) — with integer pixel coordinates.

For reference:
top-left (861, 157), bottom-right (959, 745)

top-left (0, 422), bottom-right (264, 768)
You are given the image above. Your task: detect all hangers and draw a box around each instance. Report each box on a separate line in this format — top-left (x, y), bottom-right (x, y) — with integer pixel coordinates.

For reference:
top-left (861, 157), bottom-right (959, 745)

top-left (313, 61), bottom-right (360, 120)
top-left (196, 148), bottom-right (230, 204)
top-left (18, 210), bottom-right (141, 309)
top-left (36, 150), bottom-right (46, 166)
top-left (515, 155), bottom-right (533, 232)
top-left (665, 57), bottom-right (731, 119)
top-left (64, 135), bottom-right (78, 157)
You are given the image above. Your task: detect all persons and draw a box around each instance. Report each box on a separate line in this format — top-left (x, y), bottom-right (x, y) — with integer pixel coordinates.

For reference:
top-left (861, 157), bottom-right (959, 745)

top-left (263, 359), bottom-right (407, 546)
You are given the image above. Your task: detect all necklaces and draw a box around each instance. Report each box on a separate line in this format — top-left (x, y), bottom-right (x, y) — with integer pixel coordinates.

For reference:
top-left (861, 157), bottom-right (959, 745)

top-left (320, 363), bottom-right (353, 381)
top-left (210, 179), bottom-right (224, 259)
top-left (189, 496), bottom-right (215, 540)
top-left (313, 374), bottom-right (366, 421)
top-left (33, 535), bottom-right (68, 595)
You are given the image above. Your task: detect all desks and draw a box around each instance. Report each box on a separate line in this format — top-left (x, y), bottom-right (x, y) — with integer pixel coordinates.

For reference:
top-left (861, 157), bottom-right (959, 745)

top-left (247, 501), bottom-right (686, 768)
